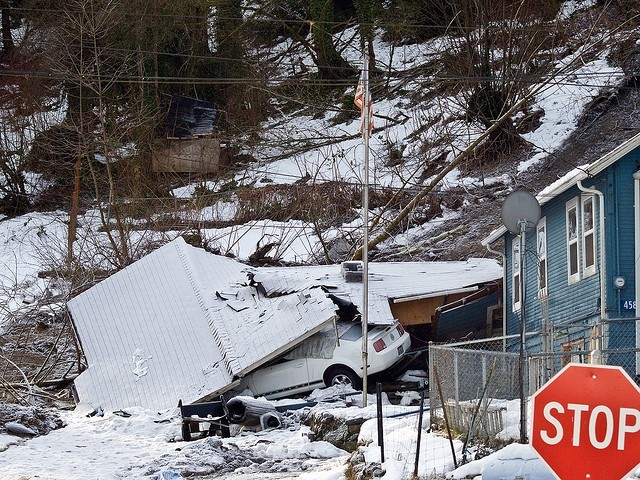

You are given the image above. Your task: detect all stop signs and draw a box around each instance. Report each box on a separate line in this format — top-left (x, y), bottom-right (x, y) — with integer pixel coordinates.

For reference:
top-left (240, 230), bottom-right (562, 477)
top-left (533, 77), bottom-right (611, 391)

top-left (529, 363), bottom-right (640, 480)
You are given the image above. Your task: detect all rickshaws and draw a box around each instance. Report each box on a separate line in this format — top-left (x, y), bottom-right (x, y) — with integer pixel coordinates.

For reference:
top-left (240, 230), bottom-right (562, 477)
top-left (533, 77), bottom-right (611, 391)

top-left (179, 395), bottom-right (230, 440)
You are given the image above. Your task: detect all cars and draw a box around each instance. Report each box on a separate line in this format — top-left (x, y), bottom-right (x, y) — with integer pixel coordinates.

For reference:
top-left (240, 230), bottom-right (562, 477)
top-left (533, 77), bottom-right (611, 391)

top-left (223, 314), bottom-right (411, 402)
